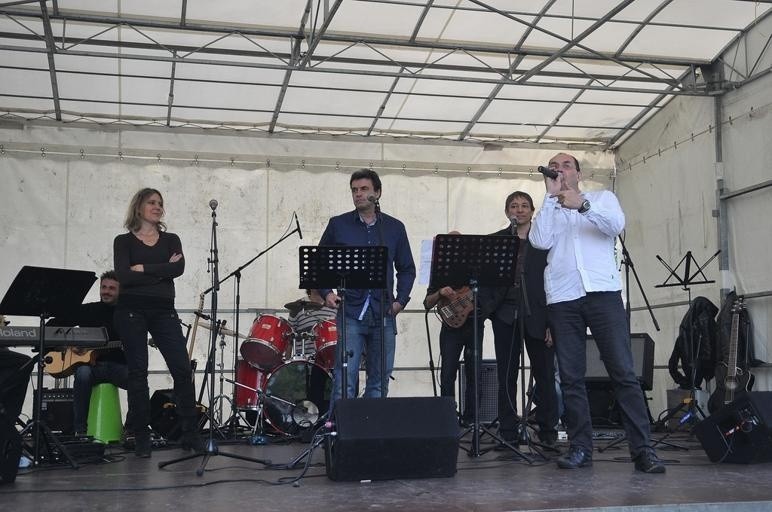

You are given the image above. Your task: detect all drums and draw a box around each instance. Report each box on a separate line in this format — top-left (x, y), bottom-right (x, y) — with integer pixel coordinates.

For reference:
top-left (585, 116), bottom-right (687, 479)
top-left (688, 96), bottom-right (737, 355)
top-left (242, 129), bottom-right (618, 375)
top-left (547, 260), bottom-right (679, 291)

top-left (311, 318), bottom-right (338, 369)
top-left (231, 359), bottom-right (268, 412)
top-left (240, 313), bottom-right (296, 373)
top-left (260, 357), bottom-right (337, 435)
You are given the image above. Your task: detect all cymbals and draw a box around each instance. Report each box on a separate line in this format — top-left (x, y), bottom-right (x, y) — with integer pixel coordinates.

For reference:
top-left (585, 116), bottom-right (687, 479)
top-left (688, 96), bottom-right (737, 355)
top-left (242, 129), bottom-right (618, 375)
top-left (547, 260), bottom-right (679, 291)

top-left (282, 296), bottom-right (326, 314)
top-left (198, 321), bottom-right (246, 340)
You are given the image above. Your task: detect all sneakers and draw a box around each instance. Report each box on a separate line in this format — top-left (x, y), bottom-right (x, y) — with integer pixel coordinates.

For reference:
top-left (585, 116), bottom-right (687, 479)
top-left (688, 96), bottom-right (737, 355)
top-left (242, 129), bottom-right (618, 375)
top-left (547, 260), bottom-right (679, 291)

top-left (134, 438), bottom-right (152, 458)
top-left (559, 447), bottom-right (592, 468)
top-left (543, 441), bottom-right (555, 451)
top-left (183, 436), bottom-right (203, 450)
top-left (635, 451), bottom-right (665, 474)
top-left (495, 439), bottom-right (519, 451)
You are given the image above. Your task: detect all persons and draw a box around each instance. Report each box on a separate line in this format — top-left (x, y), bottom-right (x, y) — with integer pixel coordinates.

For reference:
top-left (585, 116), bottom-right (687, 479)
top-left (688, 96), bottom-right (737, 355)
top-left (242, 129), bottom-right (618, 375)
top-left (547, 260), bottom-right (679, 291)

top-left (530, 153), bottom-right (666, 475)
top-left (424, 232), bottom-right (484, 427)
top-left (113, 189), bottom-right (207, 457)
top-left (314, 169), bottom-right (416, 401)
top-left (40, 271), bottom-right (168, 448)
top-left (288, 290), bottom-right (338, 358)
top-left (482, 189), bottom-right (560, 453)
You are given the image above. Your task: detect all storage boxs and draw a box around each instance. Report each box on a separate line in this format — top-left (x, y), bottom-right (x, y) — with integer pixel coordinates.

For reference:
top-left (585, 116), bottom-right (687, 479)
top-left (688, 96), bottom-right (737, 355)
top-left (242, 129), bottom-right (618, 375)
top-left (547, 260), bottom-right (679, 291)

top-left (457, 358), bottom-right (511, 423)
top-left (571, 329), bottom-right (655, 421)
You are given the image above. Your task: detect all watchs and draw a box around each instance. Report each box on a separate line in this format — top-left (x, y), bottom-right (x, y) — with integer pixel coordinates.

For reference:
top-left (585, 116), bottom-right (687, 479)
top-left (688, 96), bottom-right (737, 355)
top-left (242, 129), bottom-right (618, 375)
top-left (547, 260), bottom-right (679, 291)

top-left (577, 200), bottom-right (591, 214)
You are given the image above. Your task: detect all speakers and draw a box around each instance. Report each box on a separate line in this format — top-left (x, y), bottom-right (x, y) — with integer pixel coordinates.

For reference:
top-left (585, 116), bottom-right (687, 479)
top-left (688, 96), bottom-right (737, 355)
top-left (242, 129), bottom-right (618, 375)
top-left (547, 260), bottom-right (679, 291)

top-left (460, 359), bottom-right (500, 425)
top-left (325, 396), bottom-right (457, 481)
top-left (695, 390), bottom-right (772, 464)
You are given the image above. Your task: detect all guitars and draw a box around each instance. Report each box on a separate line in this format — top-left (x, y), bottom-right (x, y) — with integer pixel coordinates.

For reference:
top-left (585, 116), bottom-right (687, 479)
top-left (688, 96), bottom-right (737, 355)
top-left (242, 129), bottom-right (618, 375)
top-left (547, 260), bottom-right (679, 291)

top-left (706, 294), bottom-right (756, 419)
top-left (436, 281), bottom-right (477, 330)
top-left (43, 340), bottom-right (123, 380)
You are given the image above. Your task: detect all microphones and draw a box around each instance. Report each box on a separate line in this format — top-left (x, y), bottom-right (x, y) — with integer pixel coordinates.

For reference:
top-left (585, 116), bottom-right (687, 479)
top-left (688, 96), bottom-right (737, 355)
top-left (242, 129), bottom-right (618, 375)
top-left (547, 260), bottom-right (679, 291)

top-left (295, 213), bottom-right (302, 240)
top-left (367, 194), bottom-right (379, 207)
top-left (538, 166), bottom-right (558, 180)
top-left (210, 199), bottom-right (218, 210)
top-left (510, 214), bottom-right (520, 237)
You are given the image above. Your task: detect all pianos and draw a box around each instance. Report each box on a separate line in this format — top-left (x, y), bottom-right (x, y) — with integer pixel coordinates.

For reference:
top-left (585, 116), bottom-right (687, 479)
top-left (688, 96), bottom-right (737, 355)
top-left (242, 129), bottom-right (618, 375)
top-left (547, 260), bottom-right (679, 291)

top-left (0, 325), bottom-right (107, 347)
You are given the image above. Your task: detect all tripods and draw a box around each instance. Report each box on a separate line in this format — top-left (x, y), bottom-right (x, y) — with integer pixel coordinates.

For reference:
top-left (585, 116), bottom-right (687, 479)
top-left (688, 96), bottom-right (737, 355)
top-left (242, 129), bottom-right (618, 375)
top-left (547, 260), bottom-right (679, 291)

top-left (651, 283), bottom-right (703, 453)
top-left (427, 288), bottom-right (535, 464)
top-left (651, 249), bottom-right (723, 433)
top-left (494, 235), bottom-right (561, 459)
top-left (159, 211), bottom-right (271, 474)
top-left (480, 234), bottom-right (562, 460)
top-left (156, 230), bottom-right (295, 443)
top-left (1, 310), bottom-right (98, 471)
top-left (290, 295), bottom-right (393, 470)
top-left (597, 259), bottom-right (689, 452)
